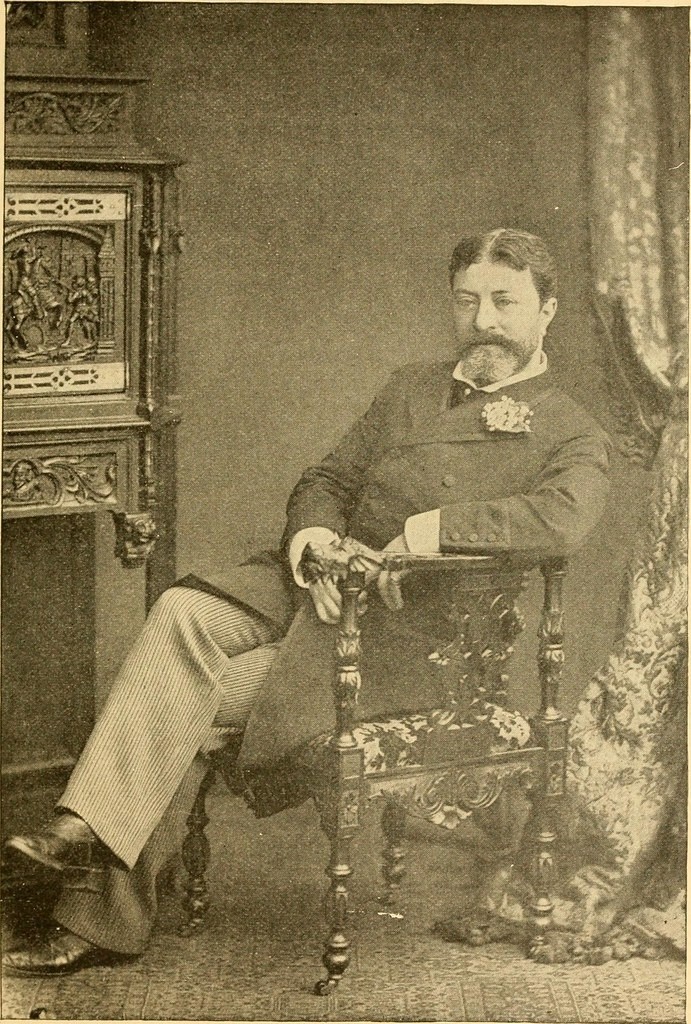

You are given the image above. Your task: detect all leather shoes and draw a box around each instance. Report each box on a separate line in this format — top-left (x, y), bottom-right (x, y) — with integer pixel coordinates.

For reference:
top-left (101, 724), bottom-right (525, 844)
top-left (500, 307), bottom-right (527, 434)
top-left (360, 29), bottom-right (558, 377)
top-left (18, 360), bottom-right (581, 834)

top-left (3, 831), bottom-right (110, 875)
top-left (0, 927), bottom-right (142, 978)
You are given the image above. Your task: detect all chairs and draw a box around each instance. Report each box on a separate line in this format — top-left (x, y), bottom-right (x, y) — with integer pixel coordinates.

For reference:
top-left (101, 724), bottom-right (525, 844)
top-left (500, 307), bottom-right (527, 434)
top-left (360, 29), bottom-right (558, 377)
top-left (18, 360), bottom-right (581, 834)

top-left (176, 547), bottom-right (567, 998)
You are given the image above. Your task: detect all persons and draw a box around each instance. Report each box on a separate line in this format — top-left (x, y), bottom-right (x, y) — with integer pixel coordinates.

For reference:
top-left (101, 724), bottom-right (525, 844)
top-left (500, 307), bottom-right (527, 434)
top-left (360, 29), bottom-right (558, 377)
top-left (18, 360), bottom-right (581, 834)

top-left (0, 227), bottom-right (612, 976)
